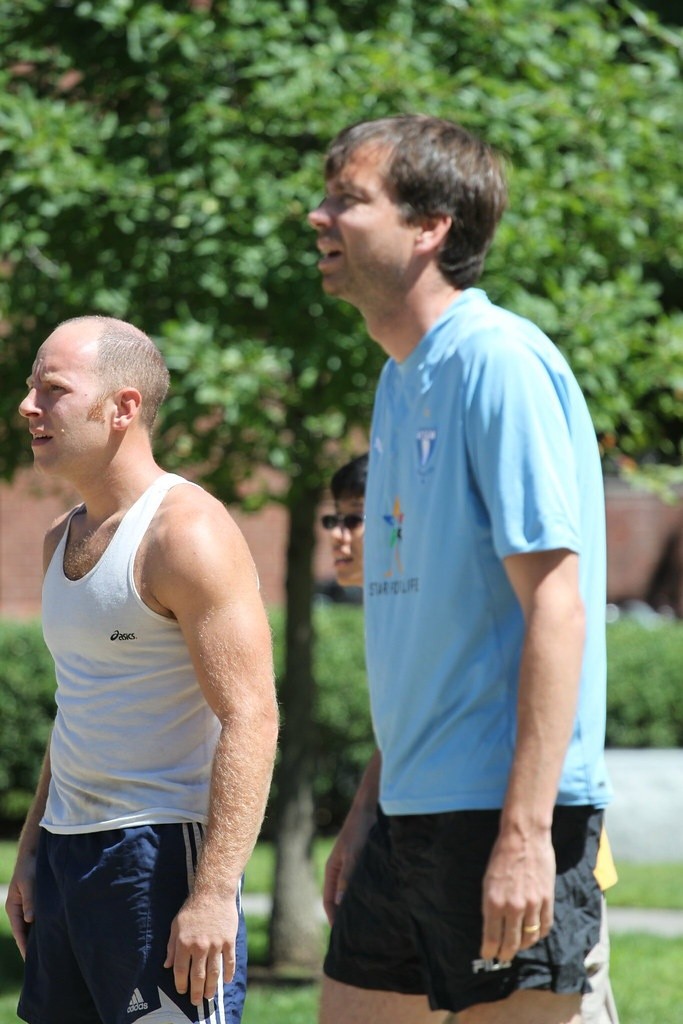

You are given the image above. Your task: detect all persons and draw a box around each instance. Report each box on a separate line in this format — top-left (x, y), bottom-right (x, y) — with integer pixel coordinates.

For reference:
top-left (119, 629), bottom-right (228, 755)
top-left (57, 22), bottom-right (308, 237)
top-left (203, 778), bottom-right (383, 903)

top-left (4, 314), bottom-right (279, 1024)
top-left (307, 113), bottom-right (610, 1024)
top-left (320, 452), bottom-right (619, 1022)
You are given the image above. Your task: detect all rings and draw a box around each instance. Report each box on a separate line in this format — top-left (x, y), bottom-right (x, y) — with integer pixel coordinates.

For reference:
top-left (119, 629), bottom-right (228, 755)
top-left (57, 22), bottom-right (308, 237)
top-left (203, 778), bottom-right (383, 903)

top-left (524, 923), bottom-right (541, 934)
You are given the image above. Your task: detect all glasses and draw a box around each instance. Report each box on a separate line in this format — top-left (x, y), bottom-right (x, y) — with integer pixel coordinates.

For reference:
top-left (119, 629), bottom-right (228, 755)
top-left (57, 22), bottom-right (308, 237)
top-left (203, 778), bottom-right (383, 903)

top-left (321, 512), bottom-right (364, 528)
